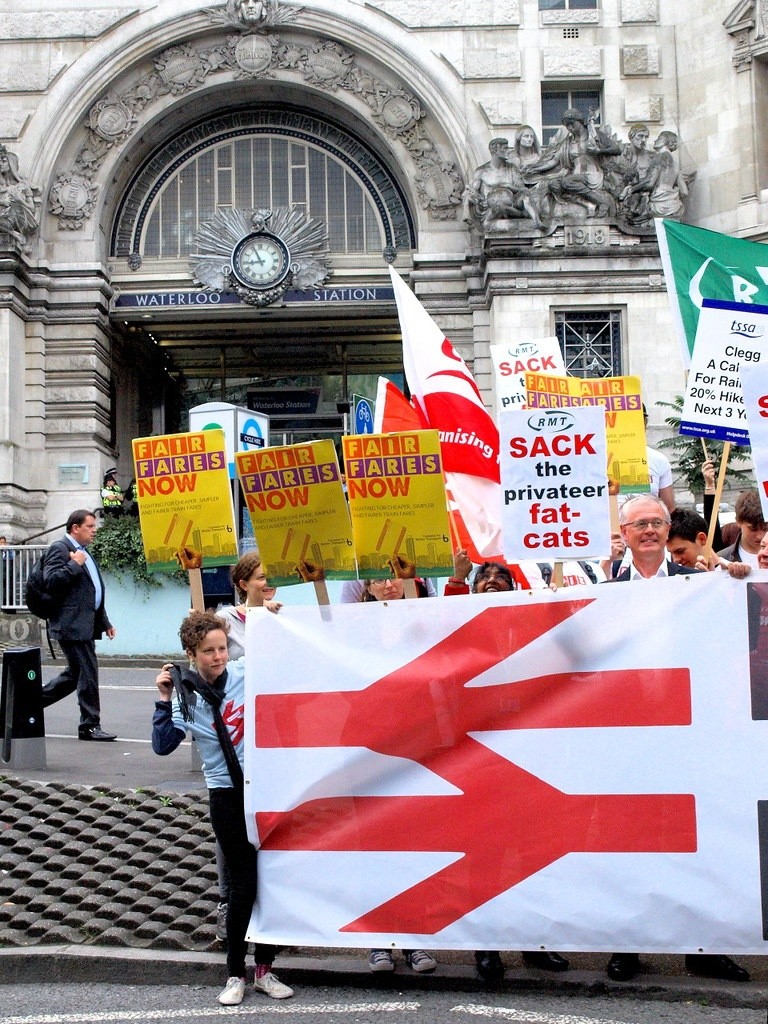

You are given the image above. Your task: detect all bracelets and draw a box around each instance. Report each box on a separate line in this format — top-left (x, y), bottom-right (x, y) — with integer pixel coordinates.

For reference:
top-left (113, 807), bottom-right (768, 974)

top-left (448, 577), bottom-right (465, 584)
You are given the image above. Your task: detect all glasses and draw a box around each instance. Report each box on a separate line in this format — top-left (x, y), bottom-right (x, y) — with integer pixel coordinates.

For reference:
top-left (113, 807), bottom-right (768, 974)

top-left (475, 572), bottom-right (512, 585)
top-left (623, 518), bottom-right (668, 529)
top-left (370, 579), bottom-right (402, 587)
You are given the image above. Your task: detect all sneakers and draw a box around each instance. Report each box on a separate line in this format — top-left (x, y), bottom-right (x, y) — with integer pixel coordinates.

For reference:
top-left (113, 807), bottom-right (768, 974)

top-left (254, 970), bottom-right (295, 998)
top-left (370, 951), bottom-right (395, 972)
top-left (216, 977), bottom-right (245, 1005)
top-left (406, 949), bottom-right (437, 971)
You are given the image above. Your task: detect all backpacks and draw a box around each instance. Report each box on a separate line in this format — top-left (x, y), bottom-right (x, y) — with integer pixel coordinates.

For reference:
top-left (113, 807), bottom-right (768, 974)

top-left (26, 554), bottom-right (58, 660)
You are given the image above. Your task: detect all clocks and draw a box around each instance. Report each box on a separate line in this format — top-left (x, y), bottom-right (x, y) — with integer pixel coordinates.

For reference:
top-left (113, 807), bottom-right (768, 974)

top-left (229, 229), bottom-right (292, 291)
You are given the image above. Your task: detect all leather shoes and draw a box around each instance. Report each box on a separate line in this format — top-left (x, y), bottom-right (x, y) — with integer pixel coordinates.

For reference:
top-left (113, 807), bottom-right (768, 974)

top-left (608, 953), bottom-right (641, 980)
top-left (79, 725), bottom-right (117, 741)
top-left (476, 958), bottom-right (502, 981)
top-left (685, 955), bottom-right (750, 980)
top-left (523, 951), bottom-right (569, 971)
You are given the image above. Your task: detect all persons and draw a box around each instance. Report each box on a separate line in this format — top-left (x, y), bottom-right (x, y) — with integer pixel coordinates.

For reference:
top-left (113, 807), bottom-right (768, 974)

top-left (125, 479), bottom-right (139, 516)
top-left (106, 468), bottom-right (123, 492)
top-left (595, 495), bottom-right (752, 985)
top-left (101, 473), bottom-right (125, 519)
top-left (362, 570), bottom-right (439, 972)
top-left (444, 546), bottom-right (568, 975)
top-left (150, 608), bottom-right (295, 1004)
top-left (214, 552), bottom-right (282, 661)
top-left (344, 402), bottom-right (767, 603)
top-left (39, 510), bottom-right (118, 740)
top-left (0, 537), bottom-right (13, 558)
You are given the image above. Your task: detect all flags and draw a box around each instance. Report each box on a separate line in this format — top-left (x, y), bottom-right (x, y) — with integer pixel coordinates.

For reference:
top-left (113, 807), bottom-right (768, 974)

top-left (389, 258), bottom-right (502, 557)
top-left (654, 216), bottom-right (768, 370)
top-left (372, 374), bottom-right (538, 591)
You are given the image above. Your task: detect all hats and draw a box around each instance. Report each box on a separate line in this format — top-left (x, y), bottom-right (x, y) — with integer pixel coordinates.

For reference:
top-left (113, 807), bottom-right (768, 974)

top-left (104, 467), bottom-right (117, 484)
